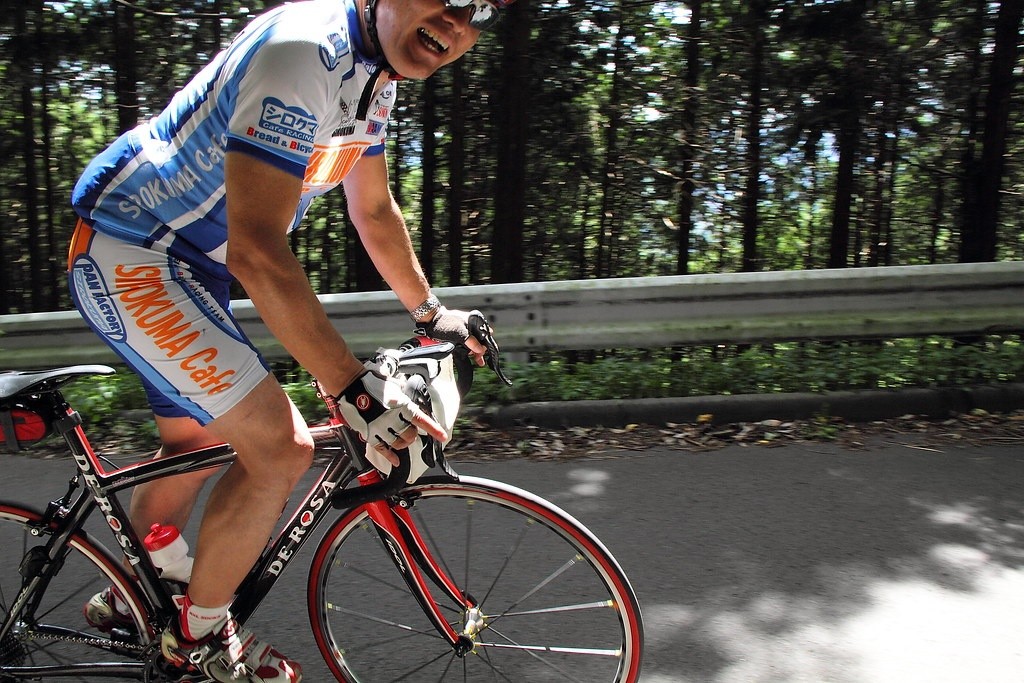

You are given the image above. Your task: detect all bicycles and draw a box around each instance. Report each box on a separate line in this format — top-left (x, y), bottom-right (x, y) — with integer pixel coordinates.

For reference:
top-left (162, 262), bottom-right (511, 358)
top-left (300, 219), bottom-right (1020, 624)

top-left (0, 315), bottom-right (645, 683)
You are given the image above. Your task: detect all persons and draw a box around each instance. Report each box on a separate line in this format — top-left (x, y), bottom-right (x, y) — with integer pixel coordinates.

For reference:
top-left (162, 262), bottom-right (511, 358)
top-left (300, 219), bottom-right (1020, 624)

top-left (69, 0), bottom-right (503, 683)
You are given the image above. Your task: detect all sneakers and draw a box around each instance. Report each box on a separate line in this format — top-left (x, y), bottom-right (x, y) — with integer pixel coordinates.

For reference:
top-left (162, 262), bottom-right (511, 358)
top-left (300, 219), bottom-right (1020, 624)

top-left (160, 595), bottom-right (303, 683)
top-left (83, 584), bottom-right (138, 634)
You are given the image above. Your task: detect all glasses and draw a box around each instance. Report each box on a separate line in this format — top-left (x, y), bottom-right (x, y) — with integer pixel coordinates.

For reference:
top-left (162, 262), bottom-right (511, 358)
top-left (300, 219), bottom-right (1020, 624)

top-left (444, 0), bottom-right (500, 30)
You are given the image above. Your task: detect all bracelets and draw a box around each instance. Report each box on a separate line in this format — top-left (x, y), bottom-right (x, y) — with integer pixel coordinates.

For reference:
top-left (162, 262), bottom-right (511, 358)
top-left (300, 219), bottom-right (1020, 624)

top-left (409, 294), bottom-right (440, 323)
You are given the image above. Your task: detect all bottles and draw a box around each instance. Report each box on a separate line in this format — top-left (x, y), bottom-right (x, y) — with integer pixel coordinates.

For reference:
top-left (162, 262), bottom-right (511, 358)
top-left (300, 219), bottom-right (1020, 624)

top-left (144, 524), bottom-right (193, 584)
